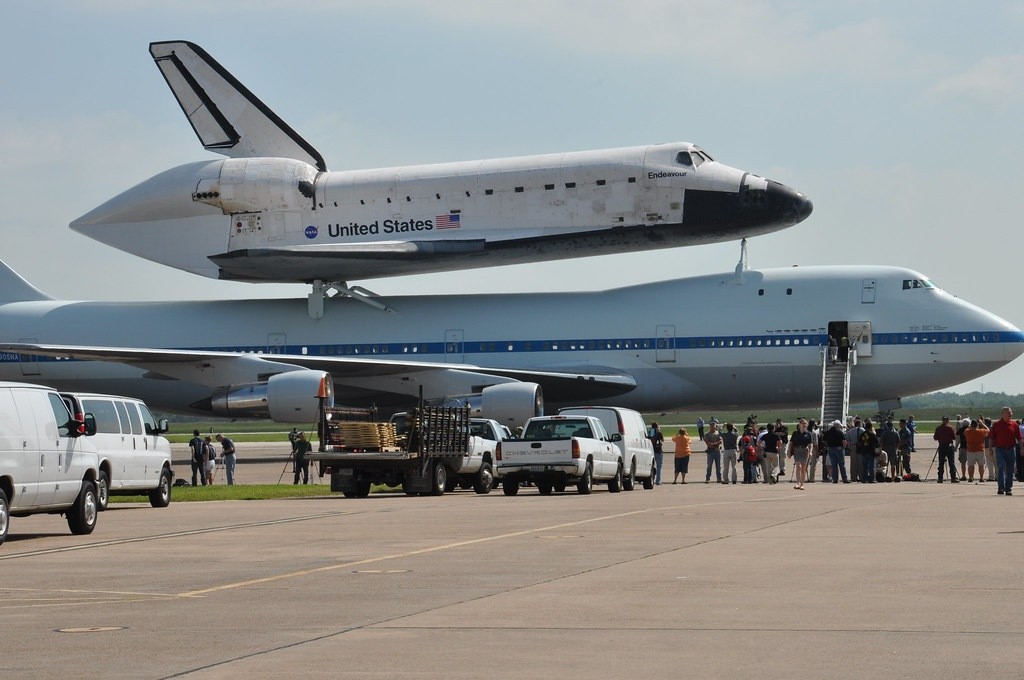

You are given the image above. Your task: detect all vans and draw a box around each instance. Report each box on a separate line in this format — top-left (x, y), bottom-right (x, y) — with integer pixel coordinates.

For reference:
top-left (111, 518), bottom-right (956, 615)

top-left (557, 406), bottom-right (656, 490)
top-left (59, 393), bottom-right (176, 512)
top-left (0, 381), bottom-right (99, 546)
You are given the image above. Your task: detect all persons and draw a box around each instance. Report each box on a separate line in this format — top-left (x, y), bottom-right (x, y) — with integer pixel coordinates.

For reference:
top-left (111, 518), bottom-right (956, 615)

top-left (719, 423), bottom-right (739, 484)
top-left (822, 419), bottom-right (852, 483)
top-left (908, 415), bottom-right (918, 452)
top-left (988, 407), bottom-right (1024, 496)
top-left (933, 416), bottom-right (991, 483)
top-left (189, 429), bottom-right (209, 487)
top-left (827, 334), bottom-right (848, 364)
top-left (748, 419), bottom-right (760, 439)
top-left (774, 418), bottom-right (788, 476)
top-left (703, 422), bottom-right (722, 483)
top-left (697, 417), bottom-right (704, 441)
top-left (290, 428), bottom-right (300, 451)
top-left (216, 434), bottom-right (236, 485)
top-left (292, 431), bottom-right (313, 484)
top-left (649, 421), bottom-right (664, 485)
top-left (1014, 417), bottom-right (1024, 482)
top-left (758, 423), bottom-right (783, 484)
top-left (738, 424), bottom-right (759, 484)
top-left (204, 436), bottom-right (216, 485)
top-left (787, 418), bottom-right (818, 490)
top-left (709, 417), bottom-right (719, 425)
top-left (815, 420), bottom-right (823, 431)
top-left (846, 417), bottom-right (912, 483)
top-left (756, 426), bottom-right (772, 483)
top-left (672, 428), bottom-right (692, 484)
top-left (955, 413), bottom-right (984, 450)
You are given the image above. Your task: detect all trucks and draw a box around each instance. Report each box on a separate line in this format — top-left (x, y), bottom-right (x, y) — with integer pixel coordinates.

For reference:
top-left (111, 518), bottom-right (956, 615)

top-left (303, 386), bottom-right (494, 497)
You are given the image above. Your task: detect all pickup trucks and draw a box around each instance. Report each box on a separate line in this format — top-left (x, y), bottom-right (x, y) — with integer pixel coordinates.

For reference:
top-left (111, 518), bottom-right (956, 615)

top-left (496, 414), bottom-right (624, 495)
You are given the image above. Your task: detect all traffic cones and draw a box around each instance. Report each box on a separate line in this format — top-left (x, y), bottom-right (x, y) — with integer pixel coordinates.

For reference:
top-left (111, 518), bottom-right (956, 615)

top-left (313, 379), bottom-right (327, 398)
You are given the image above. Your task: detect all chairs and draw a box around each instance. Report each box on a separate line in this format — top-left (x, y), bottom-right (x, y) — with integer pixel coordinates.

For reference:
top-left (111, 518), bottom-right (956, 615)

top-left (539, 429), bottom-right (551, 438)
top-left (579, 428), bottom-right (590, 437)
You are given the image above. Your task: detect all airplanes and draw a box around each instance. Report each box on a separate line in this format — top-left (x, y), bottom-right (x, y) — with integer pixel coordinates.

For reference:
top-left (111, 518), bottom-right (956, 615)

top-left (70, 41), bottom-right (812, 284)
top-left (0, 262), bottom-right (1024, 425)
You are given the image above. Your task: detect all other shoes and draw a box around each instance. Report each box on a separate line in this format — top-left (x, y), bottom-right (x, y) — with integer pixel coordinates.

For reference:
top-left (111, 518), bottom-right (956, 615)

top-left (705, 478), bottom-right (886, 490)
top-left (959, 477), bottom-right (967, 481)
top-left (1006, 489), bottom-right (1012, 495)
top-left (969, 478), bottom-right (973, 482)
top-left (937, 479), bottom-right (943, 483)
top-left (672, 481), bottom-right (677, 484)
top-left (657, 482), bottom-right (663, 485)
top-left (951, 479), bottom-right (960, 482)
top-left (986, 478), bottom-right (995, 481)
top-left (998, 489), bottom-right (1004, 494)
top-left (980, 479), bottom-right (985, 482)
top-left (681, 481), bottom-right (688, 484)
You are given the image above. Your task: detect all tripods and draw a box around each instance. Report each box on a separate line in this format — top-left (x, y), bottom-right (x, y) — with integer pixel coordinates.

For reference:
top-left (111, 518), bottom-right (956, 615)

top-left (212, 446), bottom-right (234, 481)
top-left (925, 448), bottom-right (961, 482)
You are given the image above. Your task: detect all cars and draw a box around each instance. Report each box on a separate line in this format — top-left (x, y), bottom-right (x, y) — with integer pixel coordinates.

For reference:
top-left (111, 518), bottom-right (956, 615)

top-left (469, 416), bottom-right (508, 442)
top-left (503, 425), bottom-right (515, 439)
